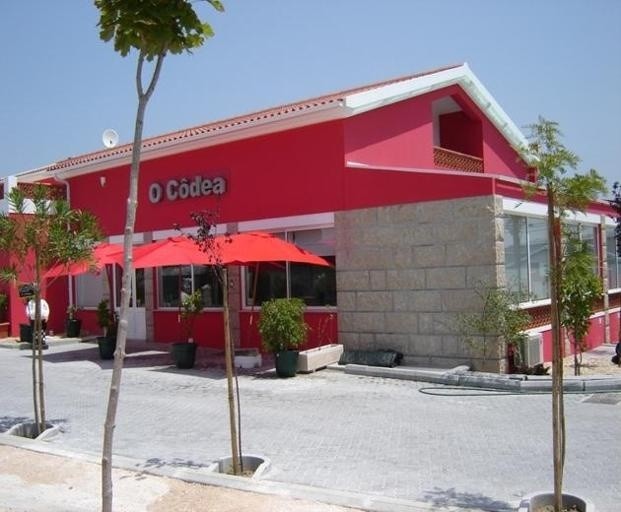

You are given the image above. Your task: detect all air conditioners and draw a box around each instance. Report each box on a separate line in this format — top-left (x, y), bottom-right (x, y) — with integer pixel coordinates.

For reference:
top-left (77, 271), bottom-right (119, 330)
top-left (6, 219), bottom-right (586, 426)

top-left (509, 331), bottom-right (551, 372)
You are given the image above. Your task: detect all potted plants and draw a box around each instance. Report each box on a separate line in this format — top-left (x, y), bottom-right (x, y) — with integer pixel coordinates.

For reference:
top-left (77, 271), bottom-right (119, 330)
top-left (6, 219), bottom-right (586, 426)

top-left (93, 296), bottom-right (128, 362)
top-left (253, 292), bottom-right (309, 379)
top-left (167, 288), bottom-right (209, 372)
top-left (62, 301), bottom-right (85, 340)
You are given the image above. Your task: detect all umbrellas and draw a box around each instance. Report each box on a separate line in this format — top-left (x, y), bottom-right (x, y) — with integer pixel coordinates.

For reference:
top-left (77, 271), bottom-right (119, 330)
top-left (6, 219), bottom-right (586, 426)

top-left (42, 230), bottom-right (334, 354)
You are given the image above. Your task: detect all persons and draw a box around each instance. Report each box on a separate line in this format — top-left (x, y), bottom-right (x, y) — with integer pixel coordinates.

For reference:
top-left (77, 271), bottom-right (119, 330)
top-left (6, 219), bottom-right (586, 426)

top-left (25, 288), bottom-right (50, 347)
